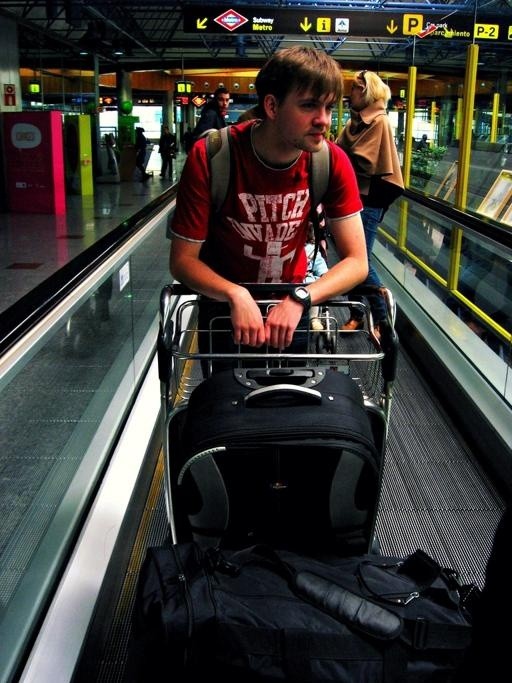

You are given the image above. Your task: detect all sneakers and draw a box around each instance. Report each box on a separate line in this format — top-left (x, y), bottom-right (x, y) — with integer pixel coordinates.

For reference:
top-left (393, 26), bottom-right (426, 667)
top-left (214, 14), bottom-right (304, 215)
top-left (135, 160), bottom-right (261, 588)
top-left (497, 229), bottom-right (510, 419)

top-left (311, 318), bottom-right (324, 330)
top-left (339, 318), bottom-right (364, 338)
top-left (367, 325), bottom-right (381, 340)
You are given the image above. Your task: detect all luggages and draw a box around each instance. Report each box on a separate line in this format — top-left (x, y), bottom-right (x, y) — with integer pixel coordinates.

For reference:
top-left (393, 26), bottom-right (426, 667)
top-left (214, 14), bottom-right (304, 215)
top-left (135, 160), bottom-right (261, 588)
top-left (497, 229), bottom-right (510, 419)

top-left (164, 366), bottom-right (388, 554)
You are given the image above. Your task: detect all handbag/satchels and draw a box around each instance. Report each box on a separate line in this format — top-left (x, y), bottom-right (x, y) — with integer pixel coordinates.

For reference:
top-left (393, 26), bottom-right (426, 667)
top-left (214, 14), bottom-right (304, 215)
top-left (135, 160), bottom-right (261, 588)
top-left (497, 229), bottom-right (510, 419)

top-left (119, 544), bottom-right (480, 682)
top-left (183, 127), bottom-right (199, 154)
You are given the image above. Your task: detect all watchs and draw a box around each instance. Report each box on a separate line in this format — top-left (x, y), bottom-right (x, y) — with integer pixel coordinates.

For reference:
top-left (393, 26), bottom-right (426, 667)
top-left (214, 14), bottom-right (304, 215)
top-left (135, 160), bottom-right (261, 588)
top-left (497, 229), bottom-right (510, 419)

top-left (289, 285), bottom-right (311, 309)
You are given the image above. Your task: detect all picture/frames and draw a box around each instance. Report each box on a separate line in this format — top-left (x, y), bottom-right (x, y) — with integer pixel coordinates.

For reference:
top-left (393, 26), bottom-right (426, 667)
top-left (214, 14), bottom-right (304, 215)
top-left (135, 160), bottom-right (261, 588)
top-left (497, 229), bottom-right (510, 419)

top-left (434, 160), bottom-right (459, 200)
top-left (476, 169), bottom-right (512, 221)
top-left (499, 203), bottom-right (512, 227)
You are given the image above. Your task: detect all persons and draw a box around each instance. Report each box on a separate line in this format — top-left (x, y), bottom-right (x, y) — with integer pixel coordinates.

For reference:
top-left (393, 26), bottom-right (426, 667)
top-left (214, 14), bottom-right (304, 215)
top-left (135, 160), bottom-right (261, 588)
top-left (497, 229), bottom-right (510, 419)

top-left (336, 69), bottom-right (405, 341)
top-left (168, 45), bottom-right (367, 376)
top-left (128, 88), bottom-right (230, 182)
top-left (416, 133), bottom-right (429, 151)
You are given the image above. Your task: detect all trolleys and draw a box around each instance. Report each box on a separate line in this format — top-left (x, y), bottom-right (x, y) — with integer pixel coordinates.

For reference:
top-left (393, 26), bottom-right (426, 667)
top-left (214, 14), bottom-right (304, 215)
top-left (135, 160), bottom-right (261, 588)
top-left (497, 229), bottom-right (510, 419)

top-left (157, 280), bottom-right (399, 543)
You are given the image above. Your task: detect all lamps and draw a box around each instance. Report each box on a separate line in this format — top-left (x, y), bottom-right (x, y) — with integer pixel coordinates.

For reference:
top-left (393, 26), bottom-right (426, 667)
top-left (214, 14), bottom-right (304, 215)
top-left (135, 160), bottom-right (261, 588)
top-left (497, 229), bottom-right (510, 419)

top-left (28, 68), bottom-right (42, 94)
top-left (399, 86), bottom-right (408, 103)
top-left (174, 53), bottom-right (192, 96)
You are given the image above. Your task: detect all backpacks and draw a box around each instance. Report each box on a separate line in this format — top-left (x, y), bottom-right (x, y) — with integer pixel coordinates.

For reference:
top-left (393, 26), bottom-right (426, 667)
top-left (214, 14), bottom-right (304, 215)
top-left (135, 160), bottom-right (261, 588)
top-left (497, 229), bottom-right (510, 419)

top-left (167, 126), bottom-right (329, 241)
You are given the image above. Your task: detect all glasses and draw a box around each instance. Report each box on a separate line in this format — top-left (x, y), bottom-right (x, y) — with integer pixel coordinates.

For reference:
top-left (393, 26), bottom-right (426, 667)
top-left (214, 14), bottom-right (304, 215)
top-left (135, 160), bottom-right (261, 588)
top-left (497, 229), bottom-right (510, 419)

top-left (359, 70), bottom-right (367, 86)
